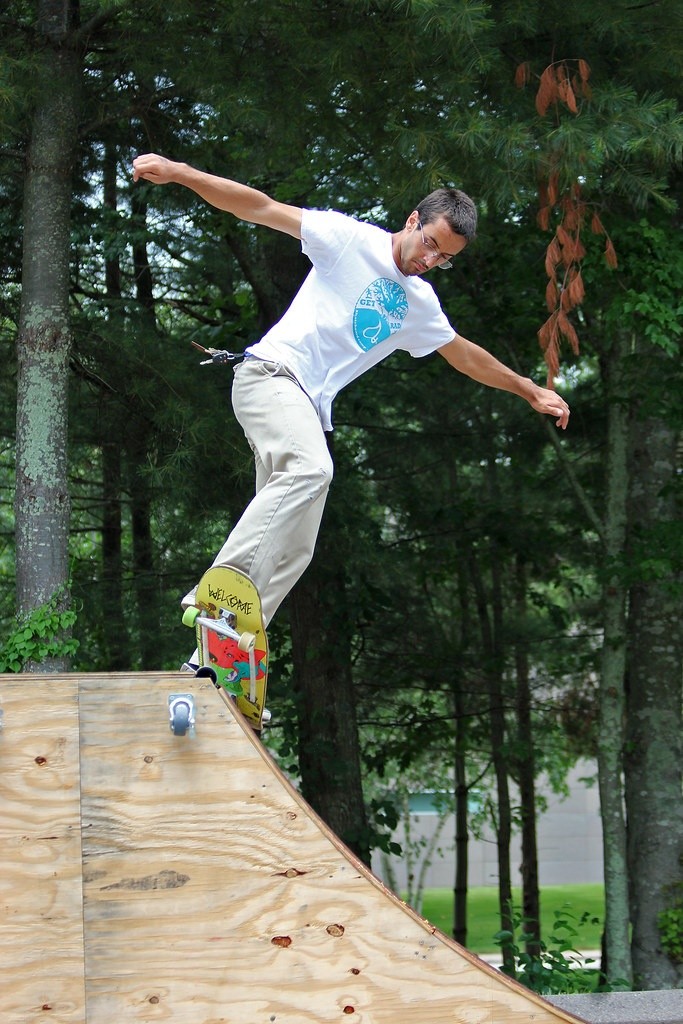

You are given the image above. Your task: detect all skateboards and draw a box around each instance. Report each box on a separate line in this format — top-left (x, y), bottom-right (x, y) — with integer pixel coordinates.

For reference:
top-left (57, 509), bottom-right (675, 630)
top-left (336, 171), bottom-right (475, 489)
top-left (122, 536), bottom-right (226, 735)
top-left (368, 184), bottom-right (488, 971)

top-left (181, 564), bottom-right (269, 732)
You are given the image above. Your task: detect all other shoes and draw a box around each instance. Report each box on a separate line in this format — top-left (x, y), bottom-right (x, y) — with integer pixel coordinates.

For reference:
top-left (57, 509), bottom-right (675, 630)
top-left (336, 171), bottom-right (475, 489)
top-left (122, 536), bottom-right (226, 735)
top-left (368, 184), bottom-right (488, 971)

top-left (181, 596), bottom-right (196, 611)
top-left (180, 662), bottom-right (271, 724)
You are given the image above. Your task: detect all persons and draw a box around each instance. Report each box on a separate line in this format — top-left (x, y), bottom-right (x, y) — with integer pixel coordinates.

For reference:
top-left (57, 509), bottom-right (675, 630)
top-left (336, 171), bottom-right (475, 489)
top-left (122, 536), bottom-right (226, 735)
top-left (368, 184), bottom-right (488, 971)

top-left (132, 154), bottom-right (569, 724)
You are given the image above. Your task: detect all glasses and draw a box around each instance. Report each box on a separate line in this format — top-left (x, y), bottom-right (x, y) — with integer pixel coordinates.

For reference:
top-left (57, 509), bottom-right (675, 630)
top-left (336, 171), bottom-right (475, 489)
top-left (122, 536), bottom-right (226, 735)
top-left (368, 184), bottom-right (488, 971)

top-left (419, 222), bottom-right (452, 270)
